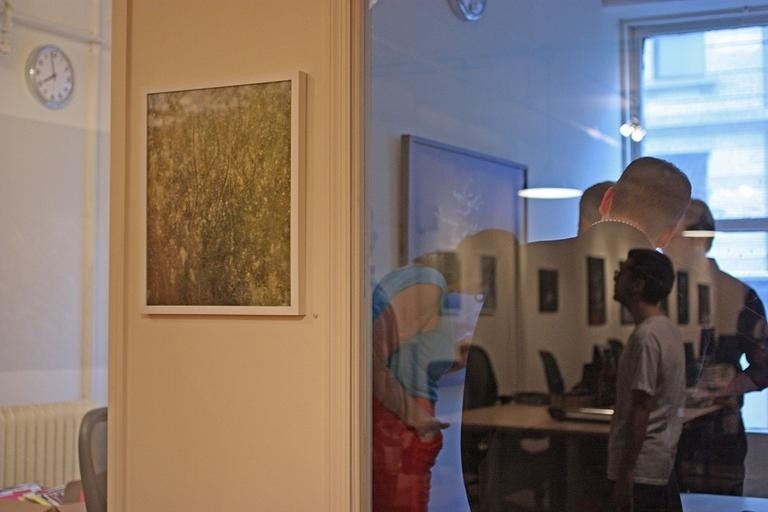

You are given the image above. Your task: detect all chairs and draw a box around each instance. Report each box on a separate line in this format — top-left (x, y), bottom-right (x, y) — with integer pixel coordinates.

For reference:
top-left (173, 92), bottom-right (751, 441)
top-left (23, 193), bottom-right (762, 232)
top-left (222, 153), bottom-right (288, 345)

top-left (456, 345), bottom-right (545, 512)
top-left (537, 349), bottom-right (566, 397)
top-left (79, 407), bottom-right (107, 512)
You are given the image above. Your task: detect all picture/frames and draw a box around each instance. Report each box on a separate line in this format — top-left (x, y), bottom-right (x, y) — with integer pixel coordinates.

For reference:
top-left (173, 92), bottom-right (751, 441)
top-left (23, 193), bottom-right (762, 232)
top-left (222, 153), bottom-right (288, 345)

top-left (399, 132), bottom-right (532, 267)
top-left (698, 284), bottom-right (710, 324)
top-left (435, 249), bottom-right (463, 313)
top-left (138, 69), bottom-right (307, 318)
top-left (619, 261), bottom-right (668, 324)
top-left (587, 256), bottom-right (606, 325)
top-left (677, 271), bottom-right (688, 324)
top-left (478, 252), bottom-right (499, 317)
top-left (538, 269), bottom-right (558, 313)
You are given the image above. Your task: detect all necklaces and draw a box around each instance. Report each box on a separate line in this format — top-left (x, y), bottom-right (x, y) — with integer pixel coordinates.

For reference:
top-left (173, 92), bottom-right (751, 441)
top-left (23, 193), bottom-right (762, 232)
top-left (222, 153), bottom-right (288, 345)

top-left (584, 219), bottom-right (656, 247)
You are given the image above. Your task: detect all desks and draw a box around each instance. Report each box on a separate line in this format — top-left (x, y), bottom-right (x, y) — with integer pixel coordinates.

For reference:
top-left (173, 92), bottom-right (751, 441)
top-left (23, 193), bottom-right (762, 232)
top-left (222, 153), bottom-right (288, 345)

top-left (397, 393), bottom-right (723, 512)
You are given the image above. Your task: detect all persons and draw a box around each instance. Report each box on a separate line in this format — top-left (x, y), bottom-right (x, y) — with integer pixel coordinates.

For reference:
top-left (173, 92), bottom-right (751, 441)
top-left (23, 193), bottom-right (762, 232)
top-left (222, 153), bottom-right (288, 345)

top-left (372, 226), bottom-right (519, 511)
top-left (577, 181), bottom-right (618, 237)
top-left (667, 201), bottom-right (767, 496)
top-left (461, 157), bottom-right (691, 512)
top-left (606, 249), bottom-right (685, 512)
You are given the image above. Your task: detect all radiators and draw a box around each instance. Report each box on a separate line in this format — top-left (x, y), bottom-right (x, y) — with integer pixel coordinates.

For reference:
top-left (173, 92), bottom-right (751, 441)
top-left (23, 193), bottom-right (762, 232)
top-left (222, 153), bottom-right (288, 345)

top-left (0, 400), bottom-right (99, 490)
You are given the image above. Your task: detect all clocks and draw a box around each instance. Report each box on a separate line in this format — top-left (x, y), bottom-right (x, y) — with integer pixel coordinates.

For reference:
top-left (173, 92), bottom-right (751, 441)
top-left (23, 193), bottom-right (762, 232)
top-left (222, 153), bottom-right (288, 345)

top-left (24, 44), bottom-right (75, 109)
top-left (448, 0), bottom-right (488, 23)
